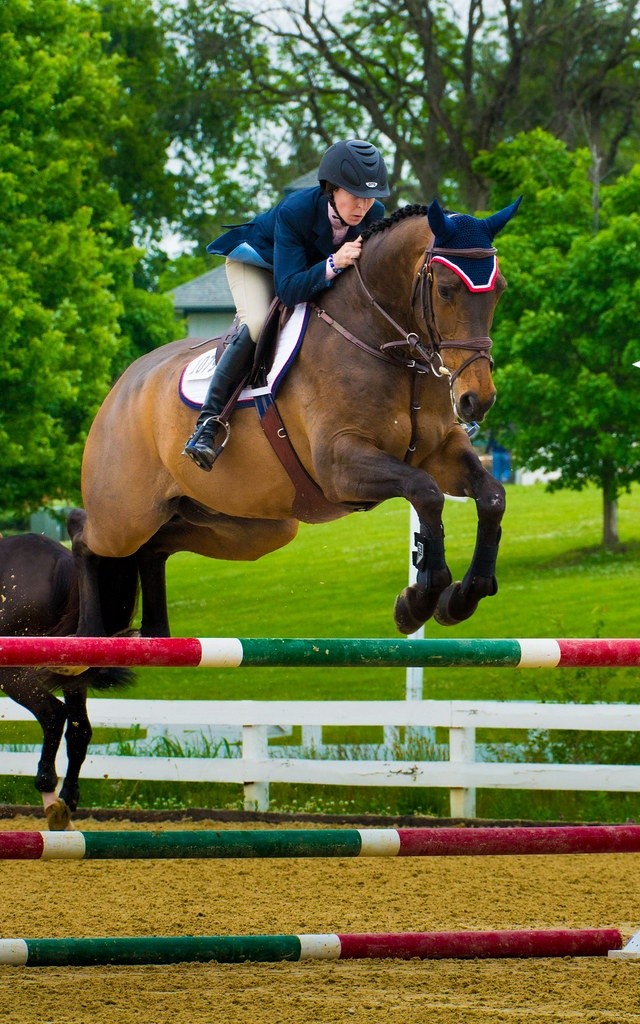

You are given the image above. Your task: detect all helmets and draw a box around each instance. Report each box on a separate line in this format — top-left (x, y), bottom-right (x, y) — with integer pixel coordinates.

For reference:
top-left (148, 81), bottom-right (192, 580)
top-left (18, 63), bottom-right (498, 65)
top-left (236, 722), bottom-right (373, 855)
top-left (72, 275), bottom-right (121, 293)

top-left (317, 140), bottom-right (390, 198)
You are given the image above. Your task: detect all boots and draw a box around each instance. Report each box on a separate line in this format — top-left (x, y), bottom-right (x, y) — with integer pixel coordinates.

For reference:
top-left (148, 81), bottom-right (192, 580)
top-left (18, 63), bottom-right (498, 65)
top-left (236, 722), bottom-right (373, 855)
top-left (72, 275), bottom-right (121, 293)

top-left (185, 323), bottom-right (258, 461)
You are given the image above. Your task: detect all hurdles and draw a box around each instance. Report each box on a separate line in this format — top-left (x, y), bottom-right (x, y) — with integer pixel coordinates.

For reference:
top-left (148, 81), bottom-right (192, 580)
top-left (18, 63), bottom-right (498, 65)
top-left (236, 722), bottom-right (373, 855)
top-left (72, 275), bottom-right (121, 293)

top-left (0, 630), bottom-right (640, 968)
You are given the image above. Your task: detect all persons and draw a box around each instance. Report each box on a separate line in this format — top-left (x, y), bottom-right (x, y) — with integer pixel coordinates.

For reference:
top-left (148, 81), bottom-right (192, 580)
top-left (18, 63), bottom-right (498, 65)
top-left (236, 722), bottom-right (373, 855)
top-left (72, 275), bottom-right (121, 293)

top-left (185, 140), bottom-right (392, 464)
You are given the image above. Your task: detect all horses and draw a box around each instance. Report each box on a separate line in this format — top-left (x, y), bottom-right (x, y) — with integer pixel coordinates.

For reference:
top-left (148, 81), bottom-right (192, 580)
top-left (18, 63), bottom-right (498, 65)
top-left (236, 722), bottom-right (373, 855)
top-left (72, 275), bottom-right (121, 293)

top-left (0, 535), bottom-right (126, 834)
top-left (82, 194), bottom-right (522, 637)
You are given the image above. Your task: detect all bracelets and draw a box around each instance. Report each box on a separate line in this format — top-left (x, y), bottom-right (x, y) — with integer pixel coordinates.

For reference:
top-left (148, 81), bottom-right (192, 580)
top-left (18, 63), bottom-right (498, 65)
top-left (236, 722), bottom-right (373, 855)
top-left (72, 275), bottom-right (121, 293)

top-left (329, 253), bottom-right (343, 273)
